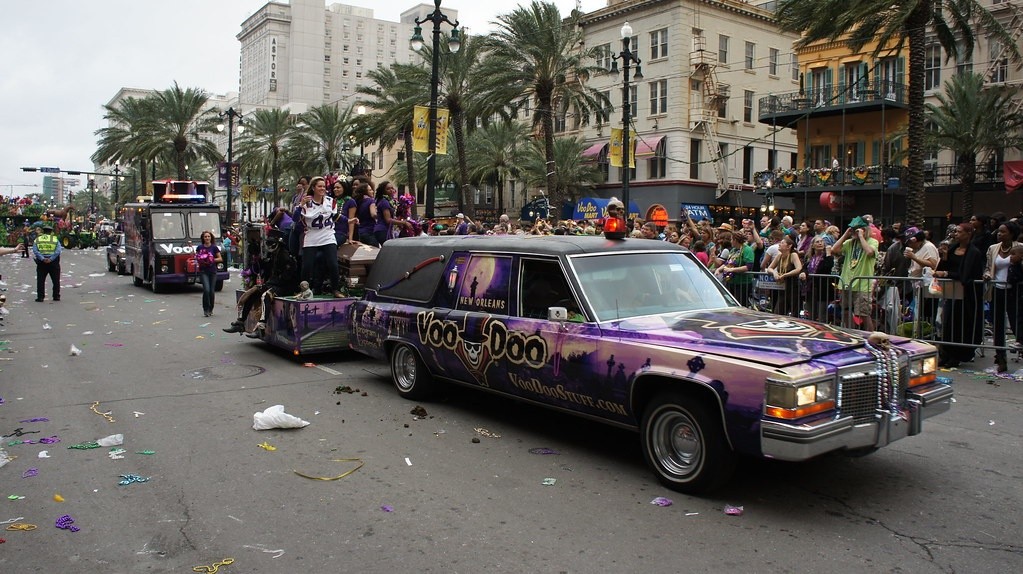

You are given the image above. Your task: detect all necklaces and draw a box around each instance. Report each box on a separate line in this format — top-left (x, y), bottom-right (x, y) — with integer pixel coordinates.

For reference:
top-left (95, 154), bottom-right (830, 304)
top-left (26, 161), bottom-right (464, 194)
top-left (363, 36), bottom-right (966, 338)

top-left (1001, 246), bottom-right (1011, 253)
top-left (335, 201), bottom-right (343, 221)
top-left (809, 253), bottom-right (822, 274)
top-left (779, 254), bottom-right (788, 274)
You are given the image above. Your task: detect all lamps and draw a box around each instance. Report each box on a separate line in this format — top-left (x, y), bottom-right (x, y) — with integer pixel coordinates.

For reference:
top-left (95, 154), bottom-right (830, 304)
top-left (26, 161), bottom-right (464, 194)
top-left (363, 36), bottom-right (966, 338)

top-left (760, 202), bottom-right (768, 212)
top-left (769, 203), bottom-right (774, 211)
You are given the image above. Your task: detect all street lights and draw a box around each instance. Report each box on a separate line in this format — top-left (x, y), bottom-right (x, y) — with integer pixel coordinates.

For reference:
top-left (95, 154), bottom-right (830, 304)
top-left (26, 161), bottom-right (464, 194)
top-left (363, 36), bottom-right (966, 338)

top-left (609, 18), bottom-right (644, 232)
top-left (110, 163), bottom-right (126, 200)
top-left (86, 177), bottom-right (99, 215)
top-left (218, 106), bottom-right (245, 229)
top-left (410, 0), bottom-right (462, 227)
top-left (67, 189), bottom-right (75, 228)
top-left (43, 195), bottom-right (58, 215)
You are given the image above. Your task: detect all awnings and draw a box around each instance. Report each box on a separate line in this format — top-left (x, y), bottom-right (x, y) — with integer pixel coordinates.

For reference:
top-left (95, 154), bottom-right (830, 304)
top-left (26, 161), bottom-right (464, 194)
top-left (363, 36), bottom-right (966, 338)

top-left (681, 203), bottom-right (713, 224)
top-left (572, 197), bottom-right (641, 220)
top-left (634, 135), bottom-right (666, 159)
top-left (582, 142), bottom-right (609, 163)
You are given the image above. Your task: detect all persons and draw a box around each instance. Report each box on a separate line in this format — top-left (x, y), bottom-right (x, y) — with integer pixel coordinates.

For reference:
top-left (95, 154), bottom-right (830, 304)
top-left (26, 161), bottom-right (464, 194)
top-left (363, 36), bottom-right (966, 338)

top-left (158, 221), bottom-right (181, 238)
top-left (33, 226), bottom-right (61, 302)
top-left (293, 281), bottom-right (313, 300)
top-left (221, 225), bottom-right (243, 271)
top-left (0, 243), bottom-right (26, 256)
top-left (22, 218), bottom-right (30, 227)
top-left (196, 231), bottom-right (222, 316)
top-left (223, 166), bottom-right (426, 340)
top-left (832, 155), bottom-right (839, 182)
top-left (0, 195), bottom-right (32, 209)
top-left (5, 218), bottom-right (15, 231)
top-left (21, 233), bottom-right (29, 258)
top-left (73, 224), bottom-right (81, 233)
top-left (419, 195), bottom-right (1023, 384)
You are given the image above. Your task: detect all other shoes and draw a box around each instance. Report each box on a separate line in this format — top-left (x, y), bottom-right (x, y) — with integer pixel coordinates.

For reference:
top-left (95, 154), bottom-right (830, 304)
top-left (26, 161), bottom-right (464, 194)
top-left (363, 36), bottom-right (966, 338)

top-left (332, 290), bottom-right (344, 298)
top-left (35, 298), bottom-right (43, 302)
top-left (53, 297), bottom-right (60, 300)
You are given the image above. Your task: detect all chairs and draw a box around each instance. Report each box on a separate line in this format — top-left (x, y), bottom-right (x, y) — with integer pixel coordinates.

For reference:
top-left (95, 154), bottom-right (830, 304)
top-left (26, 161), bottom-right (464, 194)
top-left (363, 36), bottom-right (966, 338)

top-left (525, 292), bottom-right (567, 314)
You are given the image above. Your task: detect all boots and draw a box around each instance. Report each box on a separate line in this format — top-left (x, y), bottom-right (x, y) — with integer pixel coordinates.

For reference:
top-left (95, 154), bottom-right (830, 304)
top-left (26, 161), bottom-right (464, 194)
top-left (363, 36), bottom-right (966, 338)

top-left (246, 321), bottom-right (266, 338)
top-left (222, 318), bottom-right (245, 336)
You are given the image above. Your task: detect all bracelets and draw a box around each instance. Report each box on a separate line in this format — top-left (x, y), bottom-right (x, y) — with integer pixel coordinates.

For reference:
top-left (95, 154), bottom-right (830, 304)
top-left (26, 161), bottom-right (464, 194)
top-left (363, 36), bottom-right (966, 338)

top-left (14, 248), bottom-right (16, 253)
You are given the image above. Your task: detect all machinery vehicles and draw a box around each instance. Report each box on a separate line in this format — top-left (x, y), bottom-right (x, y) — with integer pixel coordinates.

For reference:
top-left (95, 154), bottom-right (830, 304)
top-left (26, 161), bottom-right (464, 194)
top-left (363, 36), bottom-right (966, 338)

top-left (59, 227), bottom-right (100, 250)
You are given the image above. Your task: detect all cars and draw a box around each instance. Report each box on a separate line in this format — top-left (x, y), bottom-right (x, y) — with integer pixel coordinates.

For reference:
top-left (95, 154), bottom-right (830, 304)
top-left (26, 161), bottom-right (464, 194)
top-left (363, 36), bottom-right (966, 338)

top-left (347, 233), bottom-right (953, 489)
top-left (107, 233), bottom-right (129, 275)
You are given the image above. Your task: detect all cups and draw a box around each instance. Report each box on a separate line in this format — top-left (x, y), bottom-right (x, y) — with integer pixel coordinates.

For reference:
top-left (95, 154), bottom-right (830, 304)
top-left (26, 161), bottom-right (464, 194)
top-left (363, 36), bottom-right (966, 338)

top-left (306, 196), bottom-right (313, 208)
top-left (904, 247), bottom-right (913, 257)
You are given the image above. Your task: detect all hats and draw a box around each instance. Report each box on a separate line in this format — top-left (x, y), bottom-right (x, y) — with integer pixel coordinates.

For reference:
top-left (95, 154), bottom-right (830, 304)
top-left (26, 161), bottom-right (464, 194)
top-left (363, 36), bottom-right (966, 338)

top-left (337, 241), bottom-right (358, 258)
top-left (904, 227), bottom-right (920, 243)
top-left (456, 213), bottom-right (464, 218)
top-left (717, 223), bottom-right (732, 233)
top-left (43, 226), bottom-right (53, 230)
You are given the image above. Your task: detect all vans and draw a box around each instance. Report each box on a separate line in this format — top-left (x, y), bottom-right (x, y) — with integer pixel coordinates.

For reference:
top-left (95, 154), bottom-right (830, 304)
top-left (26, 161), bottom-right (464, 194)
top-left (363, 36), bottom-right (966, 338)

top-left (123, 180), bottom-right (229, 292)
